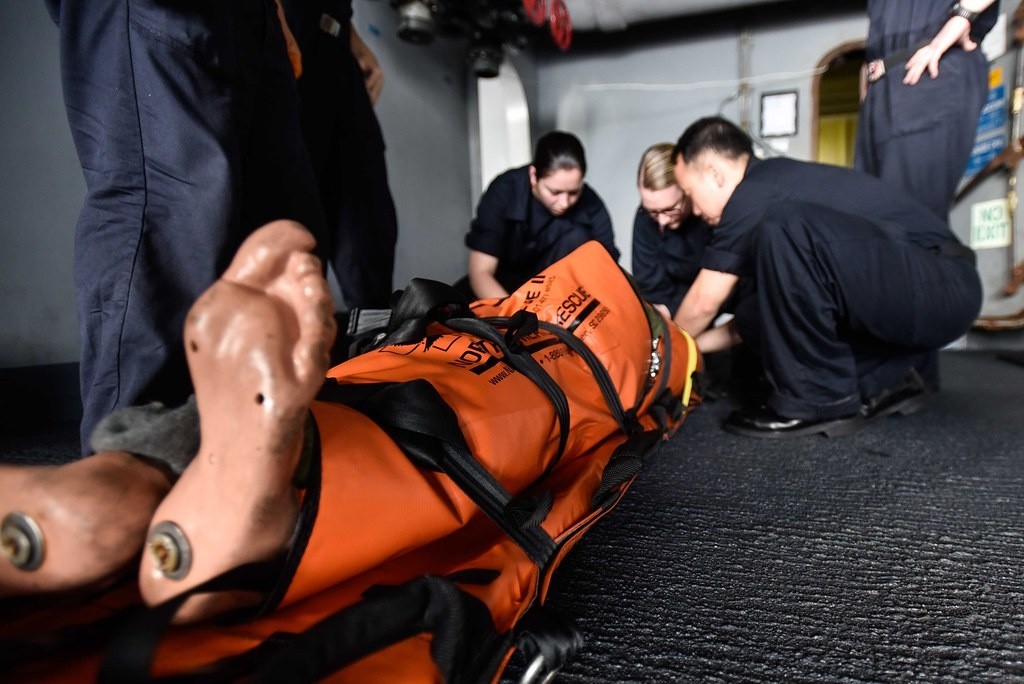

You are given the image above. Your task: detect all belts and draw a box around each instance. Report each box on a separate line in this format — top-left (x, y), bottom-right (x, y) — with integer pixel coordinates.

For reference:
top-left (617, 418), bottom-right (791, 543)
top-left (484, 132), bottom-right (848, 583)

top-left (866, 36), bottom-right (934, 84)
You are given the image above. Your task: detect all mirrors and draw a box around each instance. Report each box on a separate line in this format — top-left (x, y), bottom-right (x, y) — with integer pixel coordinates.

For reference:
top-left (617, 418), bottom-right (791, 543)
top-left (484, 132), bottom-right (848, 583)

top-left (760, 92), bottom-right (797, 138)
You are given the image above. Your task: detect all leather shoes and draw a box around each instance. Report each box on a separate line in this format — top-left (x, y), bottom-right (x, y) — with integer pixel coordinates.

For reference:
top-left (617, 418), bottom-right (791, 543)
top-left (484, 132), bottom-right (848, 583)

top-left (867, 372), bottom-right (928, 422)
top-left (718, 399), bottom-right (865, 439)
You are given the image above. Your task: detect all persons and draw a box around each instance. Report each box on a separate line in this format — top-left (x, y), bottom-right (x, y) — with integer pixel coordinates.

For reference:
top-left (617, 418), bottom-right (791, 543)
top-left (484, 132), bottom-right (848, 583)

top-left (0, 218), bottom-right (704, 623)
top-left (630, 115), bottom-right (984, 440)
top-left (451, 131), bottom-right (631, 310)
top-left (44, 0), bottom-right (401, 461)
top-left (852, 0), bottom-right (1001, 224)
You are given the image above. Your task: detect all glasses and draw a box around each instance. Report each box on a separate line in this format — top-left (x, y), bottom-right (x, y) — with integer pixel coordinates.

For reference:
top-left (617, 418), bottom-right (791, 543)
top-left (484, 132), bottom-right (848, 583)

top-left (640, 195), bottom-right (686, 219)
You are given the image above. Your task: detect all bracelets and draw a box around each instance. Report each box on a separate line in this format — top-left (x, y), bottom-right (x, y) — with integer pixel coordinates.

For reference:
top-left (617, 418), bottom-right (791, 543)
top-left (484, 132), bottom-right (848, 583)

top-left (948, 3), bottom-right (976, 22)
